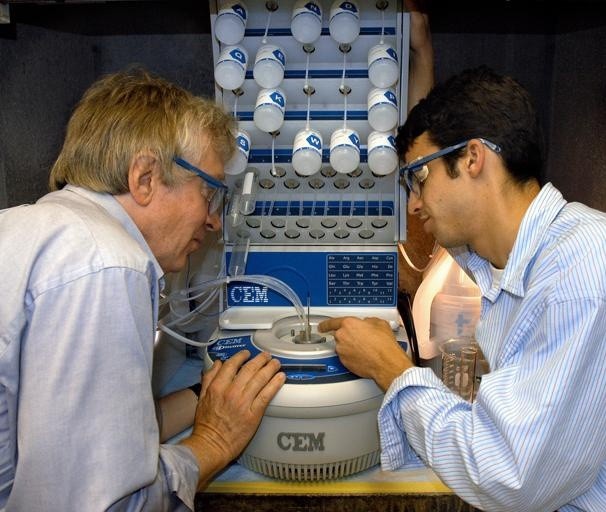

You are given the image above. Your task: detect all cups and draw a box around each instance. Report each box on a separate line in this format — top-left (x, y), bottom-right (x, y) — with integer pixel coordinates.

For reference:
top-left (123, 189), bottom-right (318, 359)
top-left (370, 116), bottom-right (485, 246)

top-left (439, 338), bottom-right (486, 403)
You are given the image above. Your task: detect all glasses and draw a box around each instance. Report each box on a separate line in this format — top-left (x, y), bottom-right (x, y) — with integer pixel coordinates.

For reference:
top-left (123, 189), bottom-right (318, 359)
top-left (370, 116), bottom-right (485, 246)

top-left (174, 156), bottom-right (229, 216)
top-left (398, 137), bottom-right (502, 201)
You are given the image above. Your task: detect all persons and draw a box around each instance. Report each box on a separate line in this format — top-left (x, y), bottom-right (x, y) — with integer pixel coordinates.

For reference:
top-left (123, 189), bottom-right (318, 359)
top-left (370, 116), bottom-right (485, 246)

top-left (314, 67), bottom-right (606, 511)
top-left (0, 60), bottom-right (290, 511)
